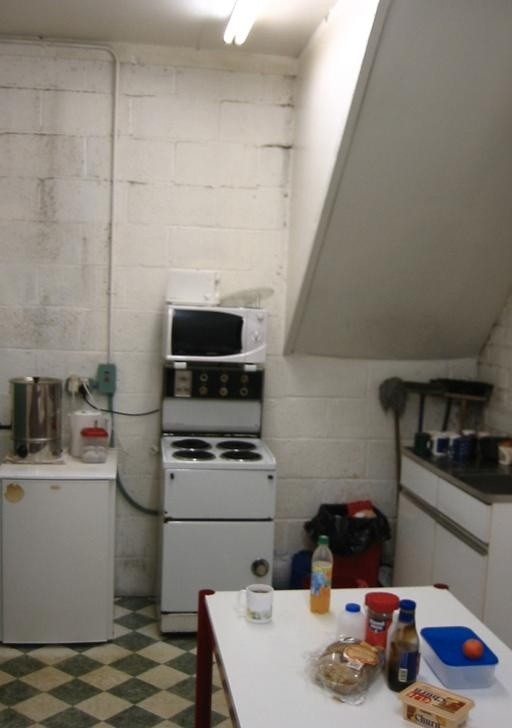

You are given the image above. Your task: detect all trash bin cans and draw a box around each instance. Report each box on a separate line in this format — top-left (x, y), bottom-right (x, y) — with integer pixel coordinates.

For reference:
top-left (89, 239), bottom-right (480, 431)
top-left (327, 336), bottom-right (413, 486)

top-left (320, 502), bottom-right (389, 589)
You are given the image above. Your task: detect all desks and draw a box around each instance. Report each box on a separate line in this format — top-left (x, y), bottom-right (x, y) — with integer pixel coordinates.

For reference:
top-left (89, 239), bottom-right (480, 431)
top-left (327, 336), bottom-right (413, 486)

top-left (194, 583), bottom-right (511, 728)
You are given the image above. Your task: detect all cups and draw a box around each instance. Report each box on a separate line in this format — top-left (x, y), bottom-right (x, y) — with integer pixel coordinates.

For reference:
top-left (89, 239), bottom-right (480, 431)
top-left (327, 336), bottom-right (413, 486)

top-left (426, 434), bottom-right (449, 458)
top-left (236, 584), bottom-right (274, 625)
top-left (366, 593), bottom-right (401, 652)
top-left (498, 442), bottom-right (512, 466)
top-left (449, 431), bottom-right (465, 457)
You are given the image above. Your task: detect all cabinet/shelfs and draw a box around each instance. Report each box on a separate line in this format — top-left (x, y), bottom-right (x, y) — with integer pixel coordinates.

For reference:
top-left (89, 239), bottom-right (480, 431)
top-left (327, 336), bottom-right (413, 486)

top-left (159, 468), bottom-right (277, 635)
top-left (392, 446), bottom-right (512, 648)
top-left (0, 446), bottom-right (119, 645)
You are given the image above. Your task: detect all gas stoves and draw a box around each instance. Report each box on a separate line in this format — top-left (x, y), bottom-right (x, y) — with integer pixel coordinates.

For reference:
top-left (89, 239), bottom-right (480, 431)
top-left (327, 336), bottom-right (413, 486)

top-left (160, 436), bottom-right (276, 470)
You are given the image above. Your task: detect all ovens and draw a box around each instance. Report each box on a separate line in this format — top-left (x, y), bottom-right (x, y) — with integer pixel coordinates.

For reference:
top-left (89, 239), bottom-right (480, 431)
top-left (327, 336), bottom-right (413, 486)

top-left (162, 307), bottom-right (268, 364)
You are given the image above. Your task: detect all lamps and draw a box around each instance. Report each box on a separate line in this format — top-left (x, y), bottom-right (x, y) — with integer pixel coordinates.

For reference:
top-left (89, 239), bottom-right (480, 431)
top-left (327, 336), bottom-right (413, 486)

top-left (223, 2), bottom-right (262, 47)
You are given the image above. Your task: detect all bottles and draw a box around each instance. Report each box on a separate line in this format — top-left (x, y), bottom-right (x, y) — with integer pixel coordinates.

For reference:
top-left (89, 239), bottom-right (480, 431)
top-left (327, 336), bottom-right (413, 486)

top-left (308, 534), bottom-right (333, 615)
top-left (386, 599), bottom-right (421, 691)
top-left (337, 602), bottom-right (367, 643)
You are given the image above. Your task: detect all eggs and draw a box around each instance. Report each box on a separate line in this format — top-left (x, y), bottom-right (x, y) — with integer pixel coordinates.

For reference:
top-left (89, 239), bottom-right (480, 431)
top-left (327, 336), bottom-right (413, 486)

top-left (461, 637), bottom-right (484, 659)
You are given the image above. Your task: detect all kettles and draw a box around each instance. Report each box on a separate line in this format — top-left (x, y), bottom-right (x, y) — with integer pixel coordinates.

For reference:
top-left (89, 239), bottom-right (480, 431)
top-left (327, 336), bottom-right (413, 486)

top-left (68, 410), bottom-right (112, 458)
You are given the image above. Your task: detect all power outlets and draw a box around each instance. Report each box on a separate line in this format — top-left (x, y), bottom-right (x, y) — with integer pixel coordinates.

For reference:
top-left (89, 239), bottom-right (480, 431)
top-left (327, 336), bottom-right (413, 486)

top-left (66, 376), bottom-right (90, 395)
